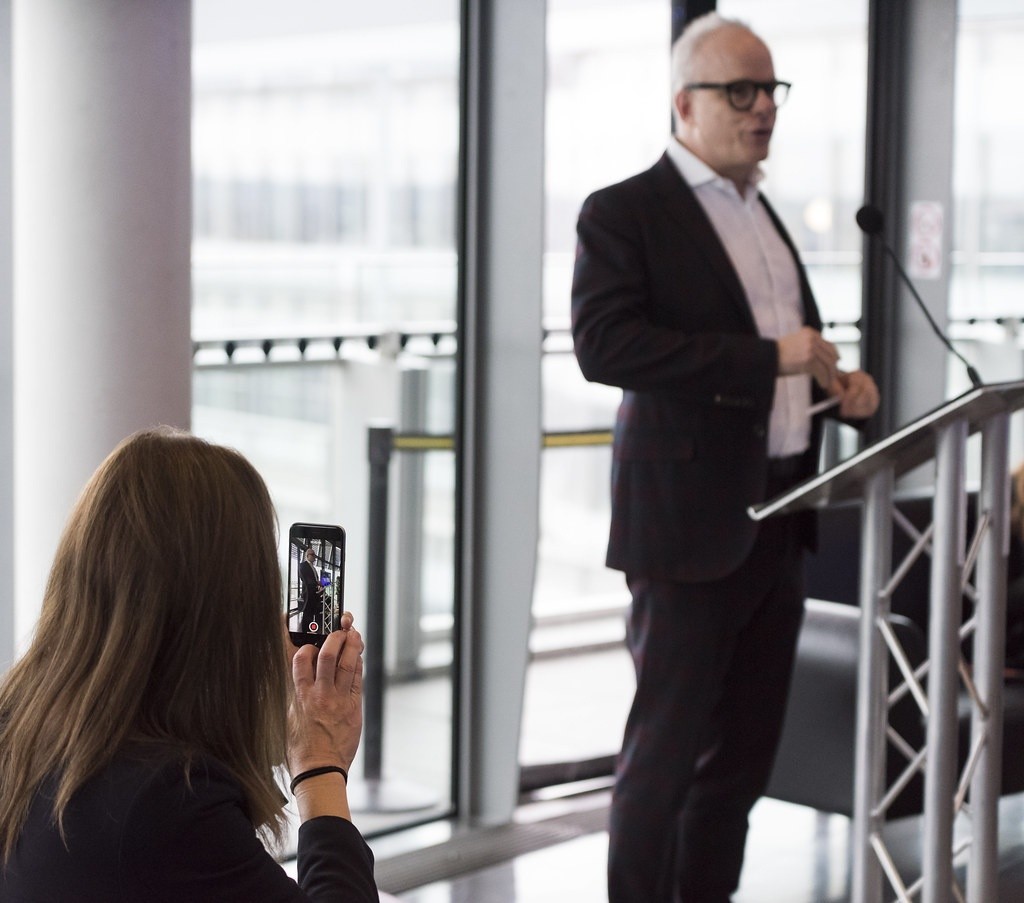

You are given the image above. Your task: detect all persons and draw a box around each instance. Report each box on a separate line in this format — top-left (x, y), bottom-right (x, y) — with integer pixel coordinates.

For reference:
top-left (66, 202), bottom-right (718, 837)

top-left (4, 424), bottom-right (381, 903)
top-left (298, 549), bottom-right (323, 634)
top-left (568, 15), bottom-right (880, 903)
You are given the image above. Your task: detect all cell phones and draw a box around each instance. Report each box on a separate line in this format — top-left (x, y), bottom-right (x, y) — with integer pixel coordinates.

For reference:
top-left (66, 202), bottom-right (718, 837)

top-left (286, 522), bottom-right (346, 650)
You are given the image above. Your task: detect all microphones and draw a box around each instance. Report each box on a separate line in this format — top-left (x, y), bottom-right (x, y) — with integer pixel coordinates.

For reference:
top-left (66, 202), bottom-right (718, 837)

top-left (854, 204), bottom-right (983, 388)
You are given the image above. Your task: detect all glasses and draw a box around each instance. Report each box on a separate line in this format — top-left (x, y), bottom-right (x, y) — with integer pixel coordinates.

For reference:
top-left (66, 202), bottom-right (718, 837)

top-left (308, 553), bottom-right (316, 557)
top-left (686, 78), bottom-right (792, 112)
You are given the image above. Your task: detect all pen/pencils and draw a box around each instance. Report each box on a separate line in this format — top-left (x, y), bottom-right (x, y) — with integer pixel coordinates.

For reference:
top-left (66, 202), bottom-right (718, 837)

top-left (799, 391), bottom-right (853, 417)
